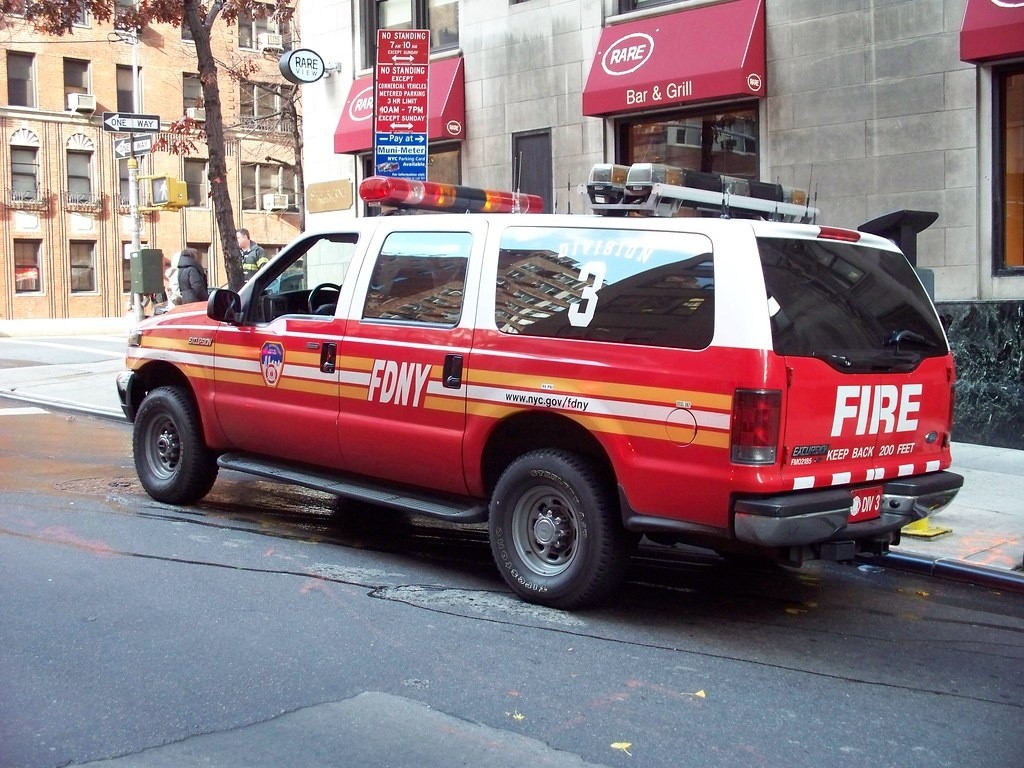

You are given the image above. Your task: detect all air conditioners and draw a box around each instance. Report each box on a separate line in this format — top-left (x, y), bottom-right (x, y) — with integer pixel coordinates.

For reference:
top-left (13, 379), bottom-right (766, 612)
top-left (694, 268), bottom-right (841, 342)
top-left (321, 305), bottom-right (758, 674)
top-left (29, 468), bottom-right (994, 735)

top-left (258, 34), bottom-right (283, 49)
top-left (263, 194), bottom-right (289, 210)
top-left (68, 92), bottom-right (96, 111)
top-left (186, 107), bottom-right (207, 122)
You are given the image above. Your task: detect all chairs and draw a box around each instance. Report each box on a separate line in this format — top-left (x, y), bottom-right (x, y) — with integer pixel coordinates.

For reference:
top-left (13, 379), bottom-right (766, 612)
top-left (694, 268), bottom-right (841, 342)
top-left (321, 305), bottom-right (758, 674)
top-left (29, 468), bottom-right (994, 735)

top-left (369, 268), bottom-right (446, 324)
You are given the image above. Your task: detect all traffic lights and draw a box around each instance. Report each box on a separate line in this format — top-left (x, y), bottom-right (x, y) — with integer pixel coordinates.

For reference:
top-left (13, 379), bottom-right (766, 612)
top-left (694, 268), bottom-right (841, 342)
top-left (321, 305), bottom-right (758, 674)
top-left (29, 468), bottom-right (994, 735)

top-left (147, 176), bottom-right (188, 209)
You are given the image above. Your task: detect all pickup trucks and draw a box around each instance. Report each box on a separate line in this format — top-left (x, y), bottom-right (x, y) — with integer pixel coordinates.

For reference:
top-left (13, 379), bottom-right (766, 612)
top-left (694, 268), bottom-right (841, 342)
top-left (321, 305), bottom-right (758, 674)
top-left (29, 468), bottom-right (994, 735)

top-left (109, 162), bottom-right (967, 611)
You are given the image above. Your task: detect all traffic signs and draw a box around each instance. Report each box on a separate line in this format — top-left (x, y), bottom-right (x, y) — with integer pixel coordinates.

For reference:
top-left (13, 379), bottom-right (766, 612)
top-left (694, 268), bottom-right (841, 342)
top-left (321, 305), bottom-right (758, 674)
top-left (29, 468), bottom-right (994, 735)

top-left (104, 111), bottom-right (160, 133)
top-left (112, 134), bottom-right (154, 158)
top-left (376, 28), bottom-right (429, 180)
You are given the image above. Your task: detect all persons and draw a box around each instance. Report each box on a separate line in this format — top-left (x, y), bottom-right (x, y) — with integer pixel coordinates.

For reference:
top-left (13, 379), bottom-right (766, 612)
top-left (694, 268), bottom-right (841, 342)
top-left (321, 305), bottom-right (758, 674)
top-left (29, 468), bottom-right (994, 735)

top-left (177, 248), bottom-right (209, 305)
top-left (165, 252), bottom-right (182, 312)
top-left (236, 229), bottom-right (269, 284)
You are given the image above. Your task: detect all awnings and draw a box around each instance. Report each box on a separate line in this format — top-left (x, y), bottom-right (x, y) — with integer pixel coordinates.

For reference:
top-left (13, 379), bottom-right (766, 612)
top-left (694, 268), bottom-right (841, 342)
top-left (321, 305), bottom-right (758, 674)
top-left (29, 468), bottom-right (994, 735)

top-left (582, 0), bottom-right (766, 116)
top-left (958, 0), bottom-right (1024, 58)
top-left (333, 58), bottom-right (466, 156)
